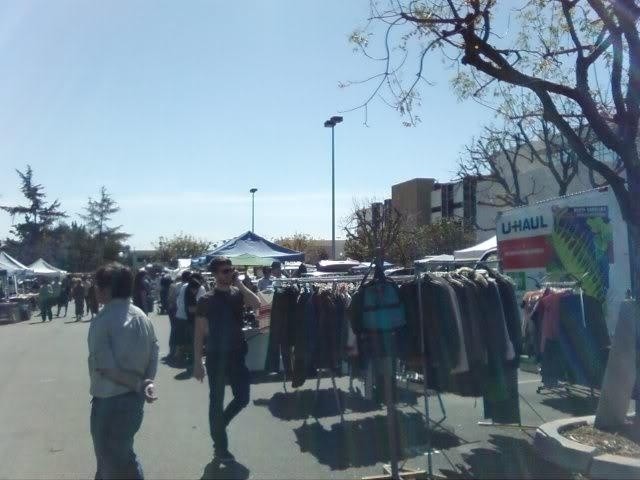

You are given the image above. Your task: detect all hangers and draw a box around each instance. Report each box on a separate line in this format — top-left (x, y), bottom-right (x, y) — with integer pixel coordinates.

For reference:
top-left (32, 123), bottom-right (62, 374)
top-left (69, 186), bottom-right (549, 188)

top-left (541, 281), bottom-right (580, 294)
top-left (424, 258), bottom-right (502, 275)
top-left (274, 277), bottom-right (364, 295)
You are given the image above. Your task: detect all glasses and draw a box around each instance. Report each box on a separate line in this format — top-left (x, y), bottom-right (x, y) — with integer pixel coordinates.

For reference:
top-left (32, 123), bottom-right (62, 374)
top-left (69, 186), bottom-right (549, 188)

top-left (218, 267), bottom-right (235, 273)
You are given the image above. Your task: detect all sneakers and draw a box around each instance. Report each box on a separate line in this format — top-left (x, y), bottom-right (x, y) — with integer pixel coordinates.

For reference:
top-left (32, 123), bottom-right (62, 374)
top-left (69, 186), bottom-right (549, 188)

top-left (213, 450), bottom-right (234, 463)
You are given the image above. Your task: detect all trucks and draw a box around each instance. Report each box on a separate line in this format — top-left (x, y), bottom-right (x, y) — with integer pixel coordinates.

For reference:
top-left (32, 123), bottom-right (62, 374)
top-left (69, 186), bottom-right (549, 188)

top-left (472, 182), bottom-right (640, 375)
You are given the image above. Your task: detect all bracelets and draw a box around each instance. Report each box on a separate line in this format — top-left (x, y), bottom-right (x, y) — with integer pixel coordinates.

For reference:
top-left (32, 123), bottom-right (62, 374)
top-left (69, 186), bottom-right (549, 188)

top-left (234, 277), bottom-right (242, 286)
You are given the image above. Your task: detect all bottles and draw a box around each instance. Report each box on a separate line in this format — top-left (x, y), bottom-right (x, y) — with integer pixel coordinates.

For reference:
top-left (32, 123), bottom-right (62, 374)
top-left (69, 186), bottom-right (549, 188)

top-left (144, 385), bottom-right (158, 399)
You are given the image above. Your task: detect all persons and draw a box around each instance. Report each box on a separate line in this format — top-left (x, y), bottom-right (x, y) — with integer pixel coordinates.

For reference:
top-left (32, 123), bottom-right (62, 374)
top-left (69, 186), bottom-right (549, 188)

top-left (166, 274), bottom-right (183, 360)
top-left (232, 262), bottom-right (307, 293)
top-left (132, 270), bottom-right (149, 318)
top-left (191, 255), bottom-right (262, 463)
top-left (174, 272), bottom-right (207, 362)
top-left (174, 270), bottom-right (192, 359)
top-left (16, 270), bottom-right (101, 321)
top-left (138, 267), bottom-right (155, 313)
top-left (184, 278), bottom-right (201, 360)
top-left (7, 274), bottom-right (16, 296)
top-left (153, 272), bottom-right (174, 316)
top-left (87, 260), bottom-right (160, 479)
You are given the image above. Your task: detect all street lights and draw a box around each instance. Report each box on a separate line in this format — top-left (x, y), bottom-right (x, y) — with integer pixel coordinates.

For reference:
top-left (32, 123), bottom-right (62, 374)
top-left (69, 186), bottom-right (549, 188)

top-left (250, 188), bottom-right (258, 234)
top-left (323, 116), bottom-right (344, 261)
